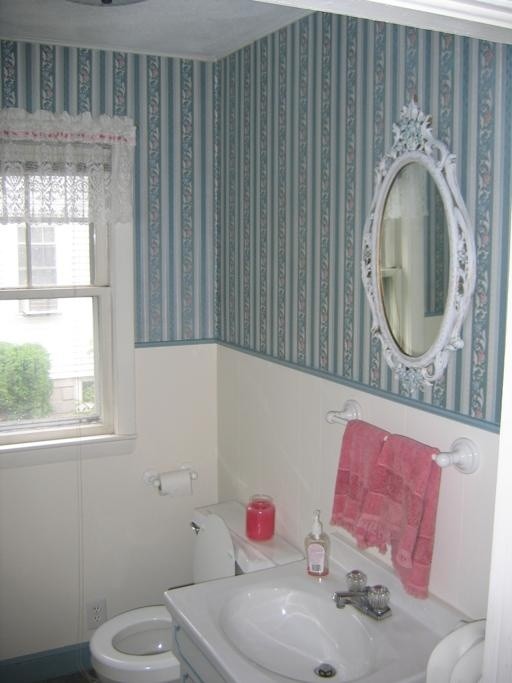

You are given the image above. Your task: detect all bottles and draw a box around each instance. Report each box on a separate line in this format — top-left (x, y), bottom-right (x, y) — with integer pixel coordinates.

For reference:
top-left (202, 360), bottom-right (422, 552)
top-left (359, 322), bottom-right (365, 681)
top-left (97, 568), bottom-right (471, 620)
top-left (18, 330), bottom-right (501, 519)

top-left (245, 493), bottom-right (276, 540)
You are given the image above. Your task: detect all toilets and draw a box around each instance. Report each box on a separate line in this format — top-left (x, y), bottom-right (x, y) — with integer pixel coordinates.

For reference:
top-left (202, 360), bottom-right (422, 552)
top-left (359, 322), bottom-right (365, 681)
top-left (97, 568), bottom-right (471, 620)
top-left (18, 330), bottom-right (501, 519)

top-left (90, 498), bottom-right (303, 681)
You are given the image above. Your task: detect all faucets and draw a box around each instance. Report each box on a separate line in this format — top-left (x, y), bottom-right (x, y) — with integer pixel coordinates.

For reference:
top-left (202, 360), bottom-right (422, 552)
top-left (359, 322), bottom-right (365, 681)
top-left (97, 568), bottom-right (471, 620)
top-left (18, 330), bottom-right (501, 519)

top-left (331, 585), bottom-right (363, 614)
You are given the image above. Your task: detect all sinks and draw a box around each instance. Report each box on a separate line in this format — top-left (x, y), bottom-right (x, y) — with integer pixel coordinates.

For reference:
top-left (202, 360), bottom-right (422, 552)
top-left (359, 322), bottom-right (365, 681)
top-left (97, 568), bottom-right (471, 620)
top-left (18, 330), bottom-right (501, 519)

top-left (164, 536), bottom-right (474, 681)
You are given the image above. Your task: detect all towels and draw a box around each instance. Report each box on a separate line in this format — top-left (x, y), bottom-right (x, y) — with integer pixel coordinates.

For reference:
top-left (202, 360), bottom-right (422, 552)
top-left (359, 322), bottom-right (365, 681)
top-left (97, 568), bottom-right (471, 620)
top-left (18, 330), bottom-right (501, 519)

top-left (329, 419), bottom-right (389, 555)
top-left (353, 434), bottom-right (442, 600)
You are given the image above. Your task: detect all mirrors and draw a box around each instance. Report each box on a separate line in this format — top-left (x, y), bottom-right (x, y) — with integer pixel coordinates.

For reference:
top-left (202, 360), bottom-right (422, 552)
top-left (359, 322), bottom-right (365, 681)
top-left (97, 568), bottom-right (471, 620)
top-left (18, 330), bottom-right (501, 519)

top-left (361, 100), bottom-right (475, 386)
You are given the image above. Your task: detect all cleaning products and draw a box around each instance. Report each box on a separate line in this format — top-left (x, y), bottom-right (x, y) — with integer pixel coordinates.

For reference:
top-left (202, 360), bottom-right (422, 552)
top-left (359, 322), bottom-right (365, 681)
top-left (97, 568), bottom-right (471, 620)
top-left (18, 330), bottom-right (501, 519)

top-left (246, 496), bottom-right (276, 544)
